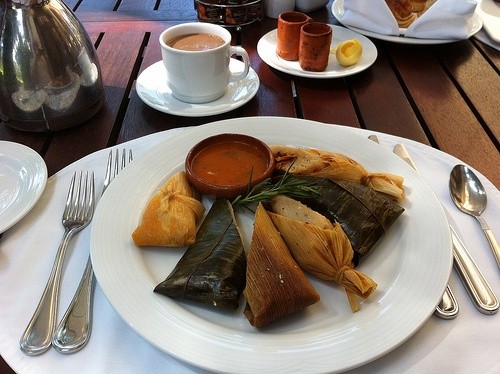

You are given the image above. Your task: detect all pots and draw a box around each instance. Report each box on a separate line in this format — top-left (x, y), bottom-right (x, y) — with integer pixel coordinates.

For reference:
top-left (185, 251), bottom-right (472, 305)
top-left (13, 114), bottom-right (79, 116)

top-left (158, 22), bottom-right (249, 104)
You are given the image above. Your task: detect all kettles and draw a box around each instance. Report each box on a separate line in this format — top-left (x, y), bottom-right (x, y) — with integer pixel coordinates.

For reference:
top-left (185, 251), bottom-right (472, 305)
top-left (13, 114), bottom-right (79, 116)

top-left (0, 0), bottom-right (104, 137)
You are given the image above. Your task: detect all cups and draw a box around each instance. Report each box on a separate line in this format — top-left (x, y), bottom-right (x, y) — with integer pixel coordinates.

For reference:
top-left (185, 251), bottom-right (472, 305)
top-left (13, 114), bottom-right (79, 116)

top-left (299, 22), bottom-right (333, 72)
top-left (277, 11), bottom-right (312, 61)
top-left (194, 0), bottom-right (260, 27)
top-left (265, 0), bottom-right (295, 18)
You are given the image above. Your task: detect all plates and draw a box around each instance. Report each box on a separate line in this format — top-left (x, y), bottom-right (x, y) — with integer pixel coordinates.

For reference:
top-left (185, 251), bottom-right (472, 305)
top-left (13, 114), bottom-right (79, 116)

top-left (0, 127), bottom-right (500, 374)
top-left (0, 139), bottom-right (47, 234)
top-left (135, 58), bottom-right (260, 117)
top-left (332, 0), bottom-right (482, 44)
top-left (481, 0), bottom-right (500, 44)
top-left (88, 115), bottom-right (452, 374)
top-left (256, 23), bottom-right (378, 80)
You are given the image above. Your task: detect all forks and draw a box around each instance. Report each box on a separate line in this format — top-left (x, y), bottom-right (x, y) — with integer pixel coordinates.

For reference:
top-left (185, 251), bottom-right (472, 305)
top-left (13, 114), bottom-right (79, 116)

top-left (19, 170), bottom-right (95, 356)
top-left (51, 148), bottom-right (131, 356)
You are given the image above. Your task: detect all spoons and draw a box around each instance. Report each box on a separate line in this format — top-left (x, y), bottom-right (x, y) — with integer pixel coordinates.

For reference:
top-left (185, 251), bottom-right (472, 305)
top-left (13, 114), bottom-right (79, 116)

top-left (449, 163), bottom-right (500, 271)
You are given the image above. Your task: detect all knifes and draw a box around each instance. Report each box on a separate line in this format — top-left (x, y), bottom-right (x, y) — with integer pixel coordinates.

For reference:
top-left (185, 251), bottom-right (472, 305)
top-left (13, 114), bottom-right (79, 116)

top-left (393, 134), bottom-right (500, 315)
top-left (366, 134), bottom-right (459, 319)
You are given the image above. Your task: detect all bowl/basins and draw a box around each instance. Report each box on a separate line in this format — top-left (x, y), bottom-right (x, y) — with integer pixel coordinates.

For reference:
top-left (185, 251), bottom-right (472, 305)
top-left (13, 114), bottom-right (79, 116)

top-left (295, 0), bottom-right (330, 12)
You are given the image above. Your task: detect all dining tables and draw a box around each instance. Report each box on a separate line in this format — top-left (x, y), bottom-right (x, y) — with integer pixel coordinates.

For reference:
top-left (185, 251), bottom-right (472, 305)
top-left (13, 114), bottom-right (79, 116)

top-left (0, 1), bottom-right (499, 374)
